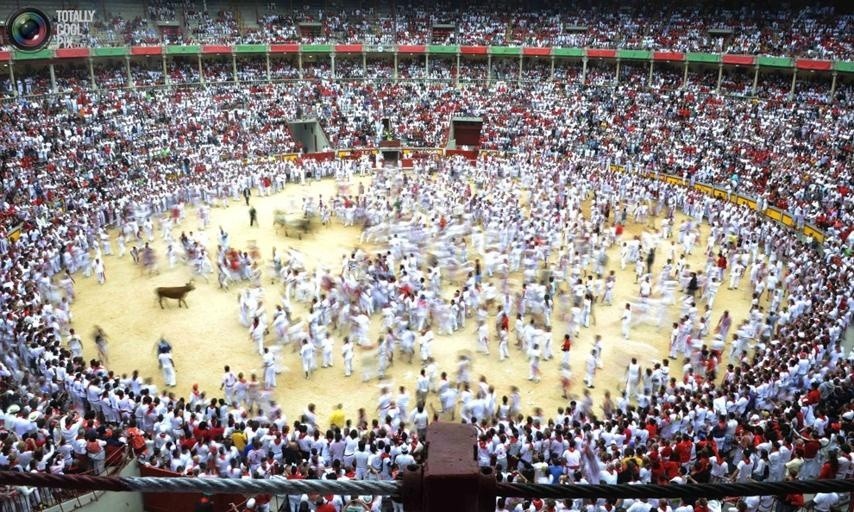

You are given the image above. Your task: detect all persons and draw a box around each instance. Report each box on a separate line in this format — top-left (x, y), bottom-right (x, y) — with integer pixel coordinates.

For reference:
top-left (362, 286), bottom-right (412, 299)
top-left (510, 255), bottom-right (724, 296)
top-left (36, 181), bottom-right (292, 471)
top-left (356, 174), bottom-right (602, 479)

top-left (0, 2), bottom-right (854, 512)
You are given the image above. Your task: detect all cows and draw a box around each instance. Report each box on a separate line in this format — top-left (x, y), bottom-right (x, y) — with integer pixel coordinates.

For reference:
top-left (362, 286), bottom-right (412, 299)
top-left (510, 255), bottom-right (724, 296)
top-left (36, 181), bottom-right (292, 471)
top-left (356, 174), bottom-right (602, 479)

top-left (154, 281), bottom-right (195, 309)
